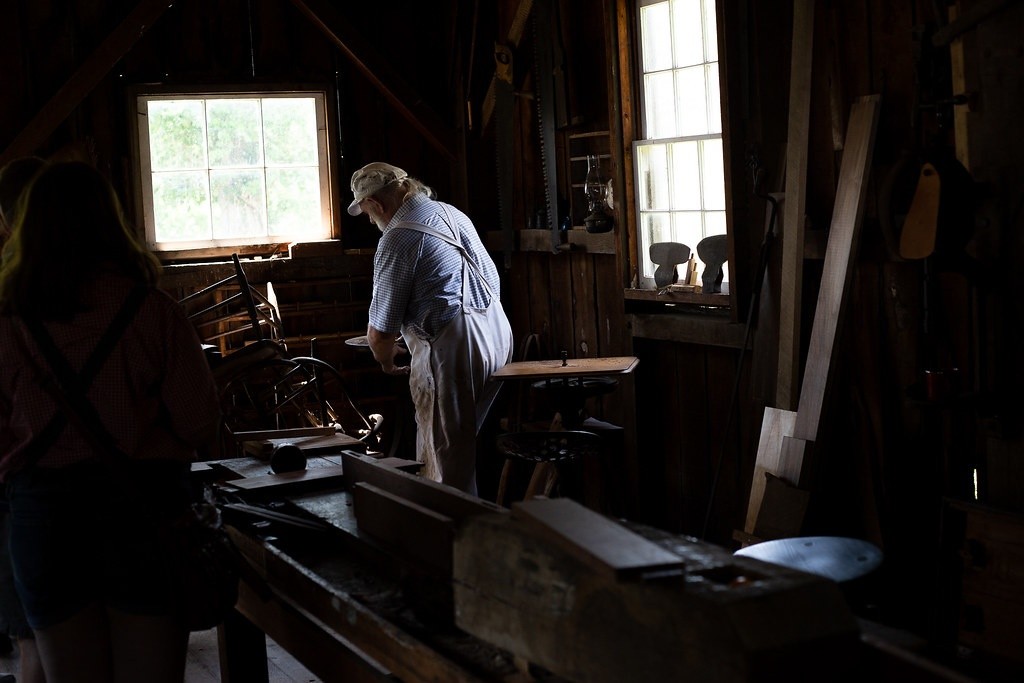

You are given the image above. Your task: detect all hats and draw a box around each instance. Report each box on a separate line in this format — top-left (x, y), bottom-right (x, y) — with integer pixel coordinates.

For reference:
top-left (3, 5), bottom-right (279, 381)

top-left (346, 163), bottom-right (407, 218)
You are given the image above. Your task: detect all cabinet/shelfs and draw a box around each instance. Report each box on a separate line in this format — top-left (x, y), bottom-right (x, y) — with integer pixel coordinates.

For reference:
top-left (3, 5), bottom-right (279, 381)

top-left (211, 270), bottom-right (408, 437)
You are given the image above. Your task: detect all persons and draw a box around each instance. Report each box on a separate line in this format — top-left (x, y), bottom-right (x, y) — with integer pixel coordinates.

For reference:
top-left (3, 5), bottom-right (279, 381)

top-left (347, 164), bottom-right (513, 498)
top-left (0, 158), bottom-right (224, 683)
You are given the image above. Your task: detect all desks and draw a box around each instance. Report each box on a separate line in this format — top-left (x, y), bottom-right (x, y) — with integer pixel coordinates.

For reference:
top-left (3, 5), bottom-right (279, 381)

top-left (185, 443), bottom-right (967, 681)
top-left (486, 356), bottom-right (640, 512)
